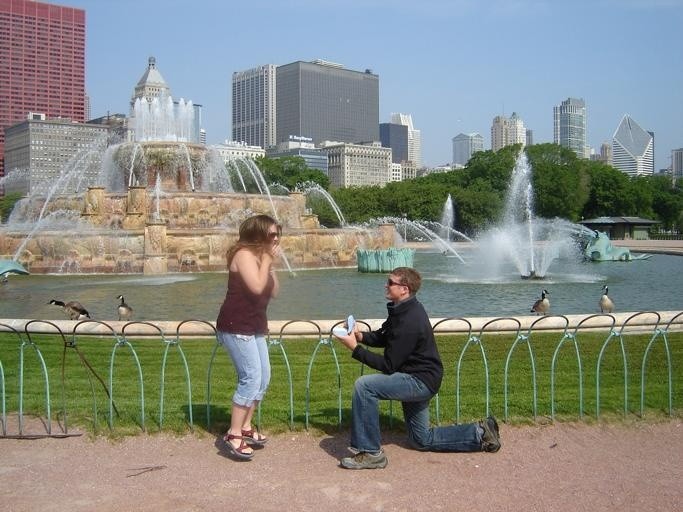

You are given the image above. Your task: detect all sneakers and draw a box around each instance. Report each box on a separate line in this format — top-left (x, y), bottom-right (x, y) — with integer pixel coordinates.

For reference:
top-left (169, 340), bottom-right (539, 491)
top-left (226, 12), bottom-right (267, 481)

top-left (479, 415), bottom-right (501, 453)
top-left (342, 448), bottom-right (388, 470)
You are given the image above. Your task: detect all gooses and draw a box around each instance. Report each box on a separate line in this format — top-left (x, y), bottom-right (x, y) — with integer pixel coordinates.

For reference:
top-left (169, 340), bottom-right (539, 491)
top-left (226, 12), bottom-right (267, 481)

top-left (598, 284), bottom-right (615, 314)
top-left (47, 298), bottom-right (91, 322)
top-left (116, 294), bottom-right (134, 321)
top-left (529, 289), bottom-right (550, 316)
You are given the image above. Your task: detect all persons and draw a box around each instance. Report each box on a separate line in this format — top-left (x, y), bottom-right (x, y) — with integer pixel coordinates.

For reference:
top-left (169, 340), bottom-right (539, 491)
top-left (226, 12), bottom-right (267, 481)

top-left (331, 266), bottom-right (501, 468)
top-left (215, 214), bottom-right (283, 461)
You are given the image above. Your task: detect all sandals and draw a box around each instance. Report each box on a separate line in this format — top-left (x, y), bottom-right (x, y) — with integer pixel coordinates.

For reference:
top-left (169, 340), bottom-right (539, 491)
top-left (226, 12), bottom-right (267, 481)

top-left (223, 430), bottom-right (269, 457)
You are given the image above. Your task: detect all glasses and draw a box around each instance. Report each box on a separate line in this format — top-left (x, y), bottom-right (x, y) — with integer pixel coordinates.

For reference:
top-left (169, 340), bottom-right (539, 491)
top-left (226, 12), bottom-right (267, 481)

top-left (388, 279), bottom-right (407, 286)
top-left (269, 232), bottom-right (280, 238)
